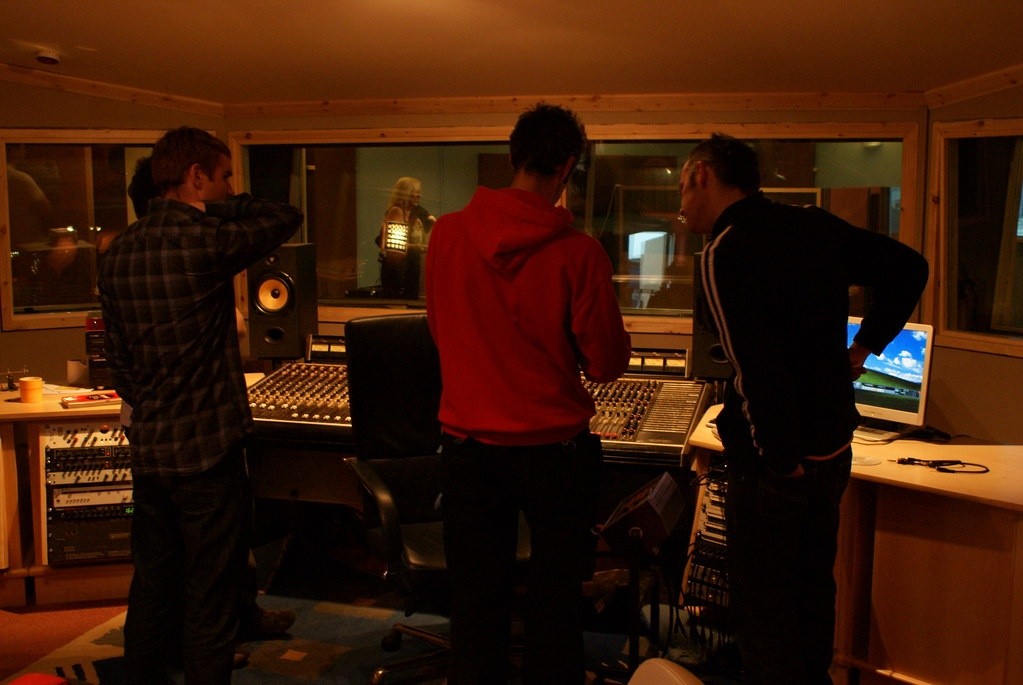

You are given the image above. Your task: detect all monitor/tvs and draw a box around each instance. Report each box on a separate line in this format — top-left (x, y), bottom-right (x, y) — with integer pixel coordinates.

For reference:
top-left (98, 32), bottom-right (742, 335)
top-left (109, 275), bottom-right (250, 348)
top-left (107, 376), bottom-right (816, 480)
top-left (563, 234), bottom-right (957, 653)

top-left (846, 315), bottom-right (935, 441)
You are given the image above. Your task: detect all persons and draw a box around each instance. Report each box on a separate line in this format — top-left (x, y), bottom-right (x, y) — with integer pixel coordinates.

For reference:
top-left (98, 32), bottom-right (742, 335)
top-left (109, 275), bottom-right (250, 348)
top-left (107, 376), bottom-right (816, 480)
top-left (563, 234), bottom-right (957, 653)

top-left (374, 176), bottom-right (436, 300)
top-left (96, 126), bottom-right (305, 685)
top-left (677, 134), bottom-right (929, 685)
top-left (424, 105), bottom-right (632, 685)
top-left (6, 143), bottom-right (127, 307)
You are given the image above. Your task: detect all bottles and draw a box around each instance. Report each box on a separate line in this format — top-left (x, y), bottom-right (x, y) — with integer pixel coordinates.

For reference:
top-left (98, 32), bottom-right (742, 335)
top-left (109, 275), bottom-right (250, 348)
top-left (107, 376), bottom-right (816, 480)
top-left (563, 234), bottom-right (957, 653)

top-left (8, 371), bottom-right (13, 389)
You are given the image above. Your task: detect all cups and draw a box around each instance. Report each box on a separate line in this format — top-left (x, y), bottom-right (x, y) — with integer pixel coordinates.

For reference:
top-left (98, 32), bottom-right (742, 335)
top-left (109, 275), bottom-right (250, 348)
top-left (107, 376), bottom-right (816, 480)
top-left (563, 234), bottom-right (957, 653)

top-left (19, 377), bottom-right (42, 403)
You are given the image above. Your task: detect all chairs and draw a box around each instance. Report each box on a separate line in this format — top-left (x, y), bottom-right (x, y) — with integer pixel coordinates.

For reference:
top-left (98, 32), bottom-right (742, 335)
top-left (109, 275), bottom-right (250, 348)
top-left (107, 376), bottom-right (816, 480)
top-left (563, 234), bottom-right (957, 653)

top-left (340, 314), bottom-right (532, 685)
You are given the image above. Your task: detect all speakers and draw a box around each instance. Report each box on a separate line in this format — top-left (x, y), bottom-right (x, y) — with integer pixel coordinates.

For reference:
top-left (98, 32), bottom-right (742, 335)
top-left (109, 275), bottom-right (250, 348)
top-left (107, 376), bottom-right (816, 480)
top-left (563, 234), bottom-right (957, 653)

top-left (246, 243), bottom-right (318, 359)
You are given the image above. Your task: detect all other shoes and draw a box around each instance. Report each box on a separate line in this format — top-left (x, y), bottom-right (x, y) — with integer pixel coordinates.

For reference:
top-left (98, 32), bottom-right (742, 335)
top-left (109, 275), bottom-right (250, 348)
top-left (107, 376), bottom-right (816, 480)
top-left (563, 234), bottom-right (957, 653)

top-left (236, 605), bottom-right (295, 639)
top-left (118, 669), bottom-right (176, 685)
top-left (233, 648), bottom-right (250, 665)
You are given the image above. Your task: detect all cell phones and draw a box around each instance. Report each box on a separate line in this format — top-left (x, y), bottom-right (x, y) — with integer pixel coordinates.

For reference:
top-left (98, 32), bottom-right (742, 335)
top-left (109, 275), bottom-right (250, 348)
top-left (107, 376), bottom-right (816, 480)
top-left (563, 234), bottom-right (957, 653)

top-left (706, 417), bottom-right (717, 428)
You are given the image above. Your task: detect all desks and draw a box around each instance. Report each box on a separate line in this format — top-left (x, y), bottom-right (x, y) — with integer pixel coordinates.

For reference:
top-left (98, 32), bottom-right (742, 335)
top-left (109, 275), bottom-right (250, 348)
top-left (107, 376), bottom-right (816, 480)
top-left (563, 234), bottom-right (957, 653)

top-left (690, 403), bottom-right (1023, 685)
